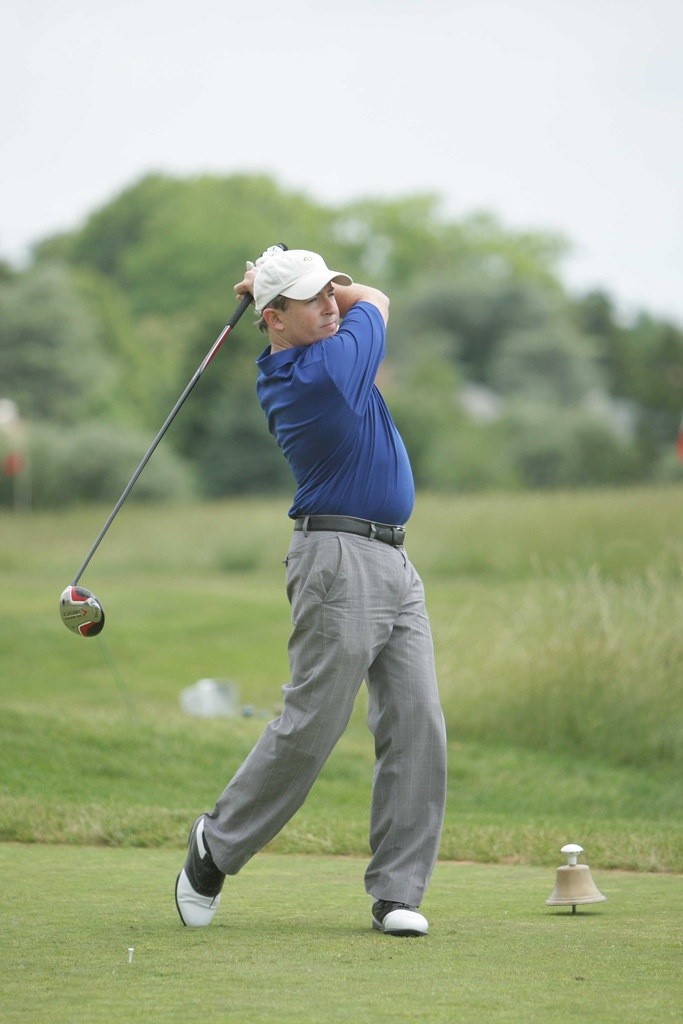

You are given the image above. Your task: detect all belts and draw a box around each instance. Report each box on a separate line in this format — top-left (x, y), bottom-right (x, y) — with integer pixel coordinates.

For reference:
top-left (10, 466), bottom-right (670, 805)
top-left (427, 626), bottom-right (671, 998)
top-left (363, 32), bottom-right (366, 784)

top-left (292, 516), bottom-right (407, 546)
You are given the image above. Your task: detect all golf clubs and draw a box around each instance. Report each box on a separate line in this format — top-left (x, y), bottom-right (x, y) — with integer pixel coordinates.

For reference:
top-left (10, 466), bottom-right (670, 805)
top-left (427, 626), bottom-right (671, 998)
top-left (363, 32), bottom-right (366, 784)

top-left (58, 244), bottom-right (289, 639)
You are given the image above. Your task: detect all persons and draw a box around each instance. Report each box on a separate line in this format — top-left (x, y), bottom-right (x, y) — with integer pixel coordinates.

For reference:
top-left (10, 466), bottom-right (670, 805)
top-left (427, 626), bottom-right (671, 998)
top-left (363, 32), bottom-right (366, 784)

top-left (176, 244), bottom-right (447, 934)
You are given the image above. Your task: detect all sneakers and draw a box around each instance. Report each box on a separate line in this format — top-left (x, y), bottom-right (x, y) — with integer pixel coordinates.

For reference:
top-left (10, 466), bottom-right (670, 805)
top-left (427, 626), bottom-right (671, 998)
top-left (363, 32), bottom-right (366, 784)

top-left (174, 810), bottom-right (227, 928)
top-left (370, 897), bottom-right (431, 935)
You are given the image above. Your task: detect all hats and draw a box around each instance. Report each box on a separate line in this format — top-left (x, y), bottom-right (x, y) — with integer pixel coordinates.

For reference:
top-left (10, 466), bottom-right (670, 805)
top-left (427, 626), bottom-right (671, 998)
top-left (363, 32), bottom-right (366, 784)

top-left (251, 249), bottom-right (353, 309)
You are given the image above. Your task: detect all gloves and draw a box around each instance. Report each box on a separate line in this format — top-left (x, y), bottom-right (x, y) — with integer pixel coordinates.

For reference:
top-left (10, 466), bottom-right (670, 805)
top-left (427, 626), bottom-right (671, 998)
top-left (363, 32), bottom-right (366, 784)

top-left (245, 246), bottom-right (284, 271)
